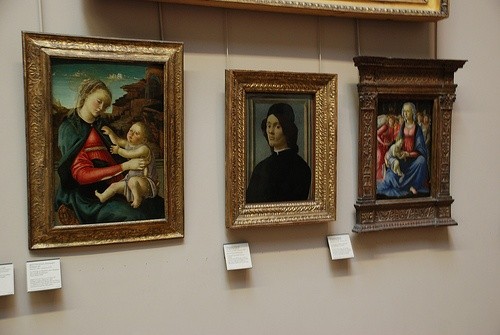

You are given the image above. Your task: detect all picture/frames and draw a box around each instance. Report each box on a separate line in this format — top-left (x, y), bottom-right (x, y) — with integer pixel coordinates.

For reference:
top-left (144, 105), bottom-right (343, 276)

top-left (225, 68), bottom-right (339, 229)
top-left (167, 0), bottom-right (449, 21)
top-left (353, 55), bottom-right (467, 233)
top-left (18, 30), bottom-right (187, 252)
